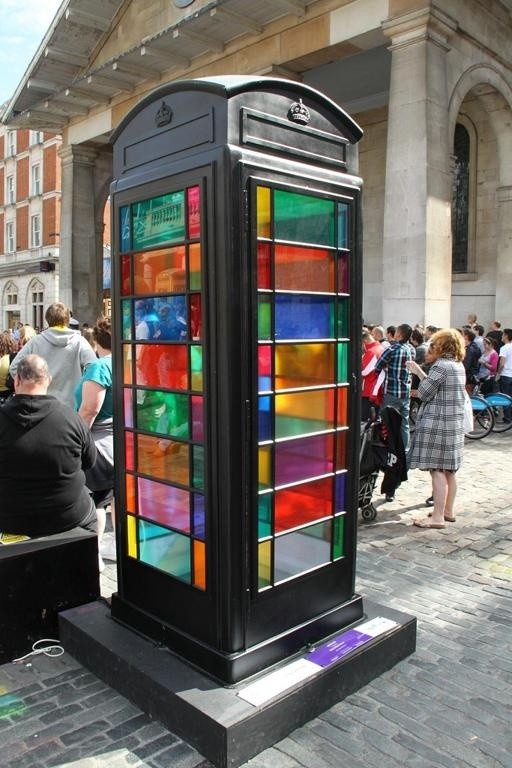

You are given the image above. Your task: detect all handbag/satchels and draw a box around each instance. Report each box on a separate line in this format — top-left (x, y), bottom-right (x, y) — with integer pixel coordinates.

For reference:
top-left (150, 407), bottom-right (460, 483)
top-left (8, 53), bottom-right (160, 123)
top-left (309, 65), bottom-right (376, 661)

top-left (462, 388), bottom-right (474, 434)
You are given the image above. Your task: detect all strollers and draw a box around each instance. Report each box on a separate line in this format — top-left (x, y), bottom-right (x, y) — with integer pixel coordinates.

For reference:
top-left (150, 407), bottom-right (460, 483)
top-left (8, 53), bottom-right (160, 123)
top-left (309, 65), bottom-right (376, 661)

top-left (358, 412), bottom-right (384, 520)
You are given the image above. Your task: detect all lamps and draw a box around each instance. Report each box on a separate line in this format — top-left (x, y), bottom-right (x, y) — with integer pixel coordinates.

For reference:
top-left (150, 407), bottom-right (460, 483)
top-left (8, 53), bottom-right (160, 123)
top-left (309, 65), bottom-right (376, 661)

top-left (40, 261), bottom-right (55, 272)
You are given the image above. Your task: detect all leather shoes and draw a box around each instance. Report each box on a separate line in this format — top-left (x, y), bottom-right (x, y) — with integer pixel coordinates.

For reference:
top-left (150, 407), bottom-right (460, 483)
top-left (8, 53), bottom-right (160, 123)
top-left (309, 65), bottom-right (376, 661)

top-left (386, 493), bottom-right (394, 501)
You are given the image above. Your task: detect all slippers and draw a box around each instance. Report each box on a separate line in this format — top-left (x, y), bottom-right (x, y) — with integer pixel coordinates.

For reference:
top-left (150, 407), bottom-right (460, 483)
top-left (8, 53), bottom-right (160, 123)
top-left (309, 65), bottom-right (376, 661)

top-left (414, 512), bottom-right (456, 528)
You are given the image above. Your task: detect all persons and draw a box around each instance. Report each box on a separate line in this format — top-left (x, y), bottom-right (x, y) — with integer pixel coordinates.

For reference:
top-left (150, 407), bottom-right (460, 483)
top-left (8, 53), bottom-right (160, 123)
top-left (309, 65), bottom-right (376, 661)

top-left (123, 300), bottom-right (187, 340)
top-left (411, 328), bottom-right (466, 528)
top-left (362, 313), bottom-right (512, 504)
top-left (0, 301), bottom-right (114, 571)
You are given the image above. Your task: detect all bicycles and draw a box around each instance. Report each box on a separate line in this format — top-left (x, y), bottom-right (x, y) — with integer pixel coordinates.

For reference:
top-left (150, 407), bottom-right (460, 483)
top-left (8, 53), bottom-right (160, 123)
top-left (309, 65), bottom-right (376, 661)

top-left (410, 374), bottom-right (495, 440)
top-left (472, 374), bottom-right (512, 433)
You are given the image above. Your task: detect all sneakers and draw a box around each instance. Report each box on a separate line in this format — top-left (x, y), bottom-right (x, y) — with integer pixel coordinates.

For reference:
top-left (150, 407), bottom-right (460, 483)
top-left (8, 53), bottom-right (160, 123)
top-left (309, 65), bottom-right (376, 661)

top-left (426, 496), bottom-right (434, 505)
